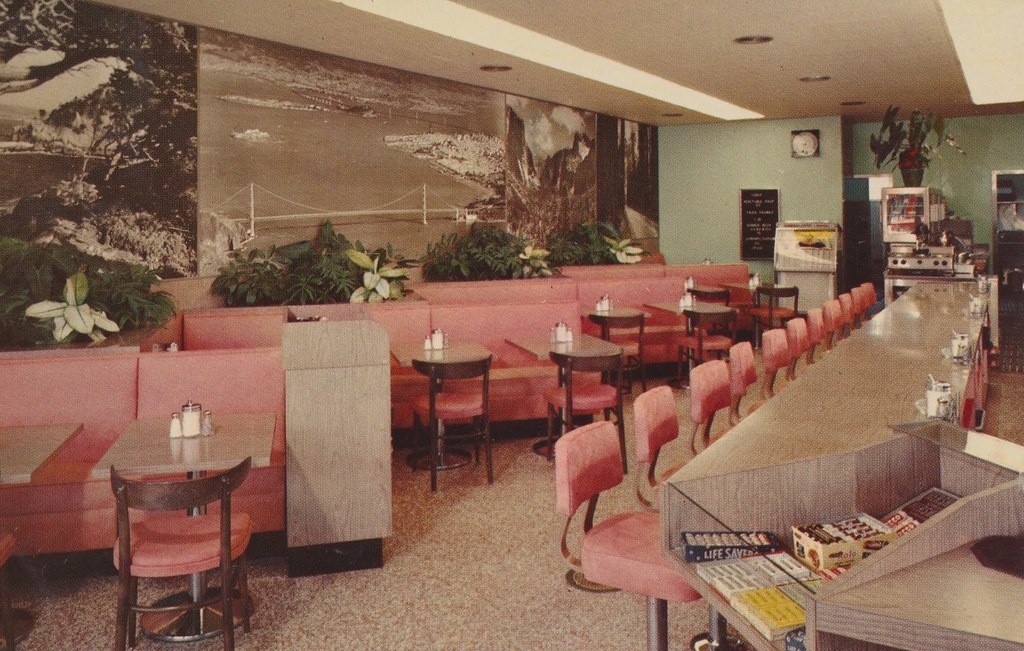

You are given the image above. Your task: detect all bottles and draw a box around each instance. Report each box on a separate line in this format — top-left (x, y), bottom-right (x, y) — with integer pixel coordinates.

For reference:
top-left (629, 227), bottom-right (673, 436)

top-left (966, 294), bottom-right (987, 318)
top-left (924, 373), bottom-right (958, 421)
top-left (679, 291), bottom-right (696, 308)
top-left (974, 273), bottom-right (989, 295)
top-left (431, 328), bottom-right (443, 350)
top-left (202, 409), bottom-right (215, 437)
top-left (169, 412), bottom-right (182, 438)
top-left (549, 319), bottom-right (573, 343)
top-left (181, 399), bottom-right (202, 437)
top-left (596, 294), bottom-right (613, 311)
top-left (424, 334), bottom-right (432, 350)
top-left (748, 272), bottom-right (762, 287)
top-left (683, 277), bottom-right (697, 289)
top-left (950, 328), bottom-right (973, 366)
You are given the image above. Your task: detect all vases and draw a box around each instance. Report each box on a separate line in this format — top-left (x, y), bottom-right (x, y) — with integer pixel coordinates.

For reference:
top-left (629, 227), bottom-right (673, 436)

top-left (899, 165), bottom-right (924, 187)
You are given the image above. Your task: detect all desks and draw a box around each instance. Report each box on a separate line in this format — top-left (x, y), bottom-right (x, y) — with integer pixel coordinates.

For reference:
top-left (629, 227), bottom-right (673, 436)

top-left (391, 336), bottom-right (493, 471)
top-left (648, 299), bottom-right (733, 391)
top-left (582, 307), bottom-right (644, 394)
top-left (1, 423), bottom-right (82, 645)
top-left (92, 411), bottom-right (278, 643)
top-left (504, 331), bottom-right (620, 457)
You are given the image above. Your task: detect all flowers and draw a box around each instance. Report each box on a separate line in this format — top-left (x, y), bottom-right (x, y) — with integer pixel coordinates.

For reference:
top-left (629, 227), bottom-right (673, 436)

top-left (867, 104), bottom-right (947, 170)
top-left (202, 219), bottom-right (418, 304)
top-left (0, 234), bottom-right (180, 346)
top-left (416, 219), bottom-right (656, 285)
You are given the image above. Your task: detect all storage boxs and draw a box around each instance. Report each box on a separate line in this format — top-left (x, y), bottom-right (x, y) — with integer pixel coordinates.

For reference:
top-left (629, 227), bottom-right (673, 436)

top-left (792, 510), bottom-right (896, 573)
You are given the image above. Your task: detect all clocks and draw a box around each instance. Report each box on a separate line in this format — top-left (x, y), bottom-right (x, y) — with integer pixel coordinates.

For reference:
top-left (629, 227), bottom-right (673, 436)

top-left (790, 129), bottom-right (820, 158)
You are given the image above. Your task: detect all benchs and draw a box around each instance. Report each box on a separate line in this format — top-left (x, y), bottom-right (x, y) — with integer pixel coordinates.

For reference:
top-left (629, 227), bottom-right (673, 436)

top-left (0, 259), bottom-right (759, 555)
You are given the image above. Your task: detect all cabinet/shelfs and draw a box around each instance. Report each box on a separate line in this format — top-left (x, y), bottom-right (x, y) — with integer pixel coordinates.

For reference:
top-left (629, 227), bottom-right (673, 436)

top-left (991, 169), bottom-right (1024, 314)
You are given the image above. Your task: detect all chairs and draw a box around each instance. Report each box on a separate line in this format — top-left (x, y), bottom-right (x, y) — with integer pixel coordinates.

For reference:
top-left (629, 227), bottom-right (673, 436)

top-left (108, 275), bottom-right (878, 651)
